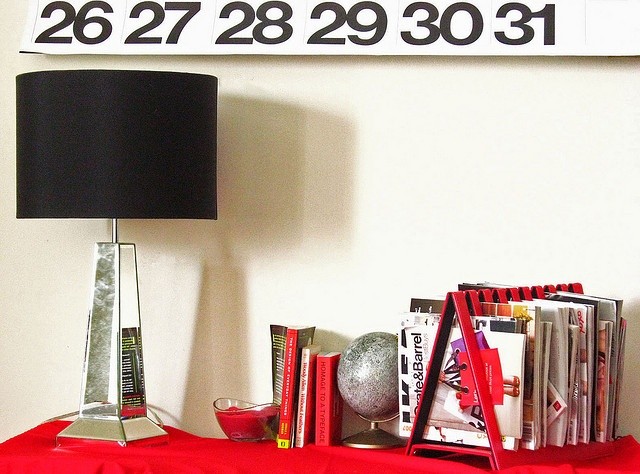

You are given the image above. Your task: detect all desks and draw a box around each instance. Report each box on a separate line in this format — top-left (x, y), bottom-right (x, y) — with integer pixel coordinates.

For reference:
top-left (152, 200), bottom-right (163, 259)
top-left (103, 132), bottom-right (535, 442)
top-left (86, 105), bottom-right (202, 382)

top-left (1, 419), bottom-right (640, 474)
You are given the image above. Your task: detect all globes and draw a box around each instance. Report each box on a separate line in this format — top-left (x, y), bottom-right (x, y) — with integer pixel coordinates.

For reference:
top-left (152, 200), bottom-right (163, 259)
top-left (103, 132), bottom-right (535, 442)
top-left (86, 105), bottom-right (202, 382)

top-left (336, 333), bottom-right (406, 450)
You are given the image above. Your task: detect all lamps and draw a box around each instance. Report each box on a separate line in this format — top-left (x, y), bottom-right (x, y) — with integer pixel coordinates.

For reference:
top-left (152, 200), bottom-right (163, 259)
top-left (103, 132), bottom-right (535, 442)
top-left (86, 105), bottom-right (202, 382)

top-left (16, 70), bottom-right (218, 449)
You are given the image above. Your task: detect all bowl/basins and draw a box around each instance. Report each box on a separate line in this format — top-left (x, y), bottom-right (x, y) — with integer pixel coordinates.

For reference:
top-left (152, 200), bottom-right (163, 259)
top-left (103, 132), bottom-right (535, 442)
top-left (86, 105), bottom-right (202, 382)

top-left (214, 397), bottom-right (279, 442)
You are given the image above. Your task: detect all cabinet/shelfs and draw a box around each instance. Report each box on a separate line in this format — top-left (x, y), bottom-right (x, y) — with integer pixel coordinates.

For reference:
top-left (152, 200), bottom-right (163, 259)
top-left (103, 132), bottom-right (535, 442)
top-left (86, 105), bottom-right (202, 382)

top-left (405, 282), bottom-right (616, 472)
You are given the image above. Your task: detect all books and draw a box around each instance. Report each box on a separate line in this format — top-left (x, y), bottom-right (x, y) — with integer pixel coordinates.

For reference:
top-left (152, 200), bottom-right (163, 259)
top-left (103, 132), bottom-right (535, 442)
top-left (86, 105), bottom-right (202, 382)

top-left (314, 351), bottom-right (344, 446)
top-left (397, 281), bottom-right (627, 451)
top-left (293, 344), bottom-right (320, 448)
top-left (270, 324), bottom-right (316, 448)
